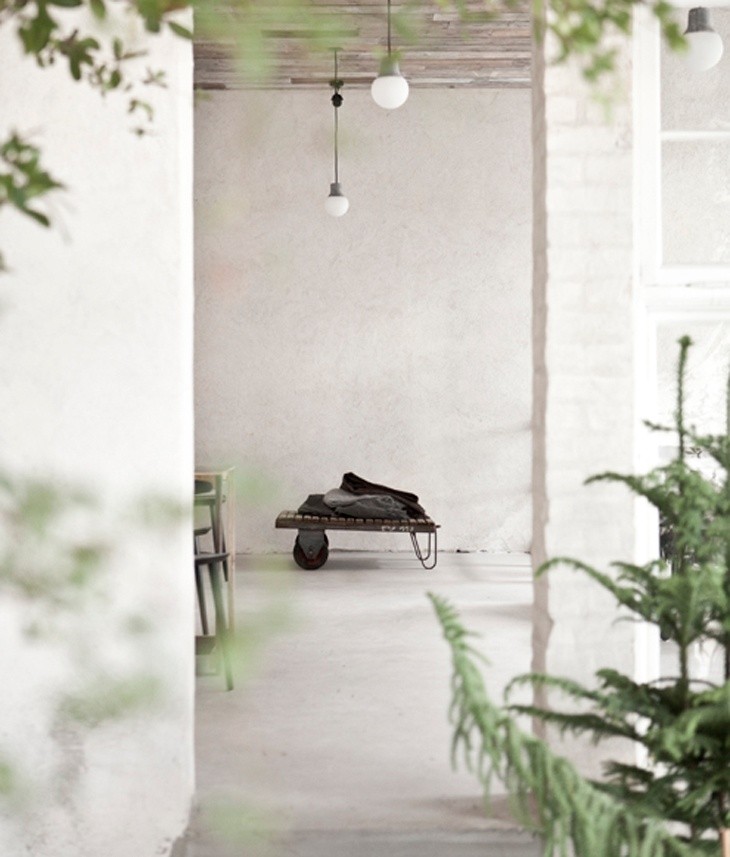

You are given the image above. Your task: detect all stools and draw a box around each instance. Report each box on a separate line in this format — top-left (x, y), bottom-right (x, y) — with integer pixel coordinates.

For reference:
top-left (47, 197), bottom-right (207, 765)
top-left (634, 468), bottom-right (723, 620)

top-left (195, 524), bottom-right (213, 637)
top-left (195, 550), bottom-right (235, 691)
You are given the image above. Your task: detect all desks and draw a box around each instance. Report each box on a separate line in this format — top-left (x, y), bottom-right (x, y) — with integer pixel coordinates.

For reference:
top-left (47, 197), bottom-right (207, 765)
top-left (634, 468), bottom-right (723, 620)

top-left (195, 465), bottom-right (237, 626)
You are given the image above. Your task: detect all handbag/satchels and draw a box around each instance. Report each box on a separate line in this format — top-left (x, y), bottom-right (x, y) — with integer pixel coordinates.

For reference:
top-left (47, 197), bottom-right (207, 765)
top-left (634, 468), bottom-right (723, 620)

top-left (295, 469), bottom-right (429, 519)
